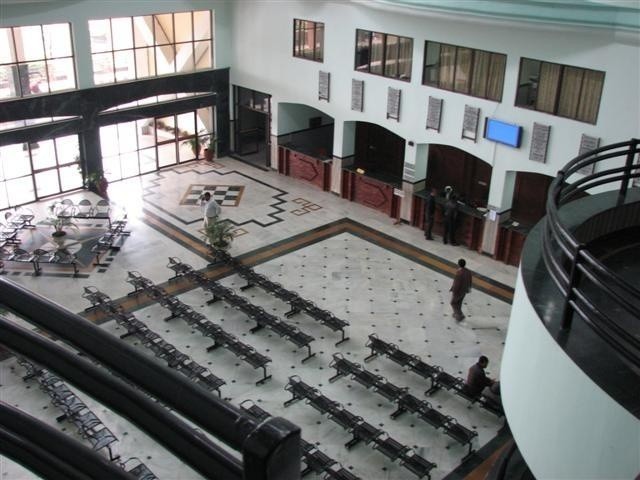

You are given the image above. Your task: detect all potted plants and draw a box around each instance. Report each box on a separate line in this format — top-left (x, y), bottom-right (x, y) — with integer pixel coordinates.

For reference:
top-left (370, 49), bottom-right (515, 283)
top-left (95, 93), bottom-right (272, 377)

top-left (182, 131), bottom-right (221, 162)
top-left (93, 169), bottom-right (109, 196)
top-left (201, 221), bottom-right (236, 250)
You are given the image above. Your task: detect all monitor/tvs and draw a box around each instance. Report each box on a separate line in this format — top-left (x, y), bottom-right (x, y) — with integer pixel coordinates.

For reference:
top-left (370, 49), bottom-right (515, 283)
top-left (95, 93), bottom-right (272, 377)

top-left (483, 117), bottom-right (522, 148)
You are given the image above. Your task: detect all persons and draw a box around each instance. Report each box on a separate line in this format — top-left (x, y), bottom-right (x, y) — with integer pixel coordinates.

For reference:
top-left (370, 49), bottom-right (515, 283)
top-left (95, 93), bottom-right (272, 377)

top-left (439, 184), bottom-right (455, 200)
top-left (423, 185), bottom-right (437, 242)
top-left (201, 192), bottom-right (221, 244)
top-left (442, 192), bottom-right (461, 247)
top-left (467, 355), bottom-right (493, 394)
top-left (472, 379), bottom-right (501, 412)
top-left (447, 258), bottom-right (473, 324)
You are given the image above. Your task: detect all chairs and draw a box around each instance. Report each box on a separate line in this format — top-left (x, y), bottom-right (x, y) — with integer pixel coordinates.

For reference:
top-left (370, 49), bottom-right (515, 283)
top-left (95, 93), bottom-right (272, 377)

top-left (1, 197), bottom-right (127, 276)
top-left (237, 331), bottom-right (504, 479)
top-left (80, 246), bottom-right (350, 401)
top-left (10, 355), bottom-right (156, 479)
